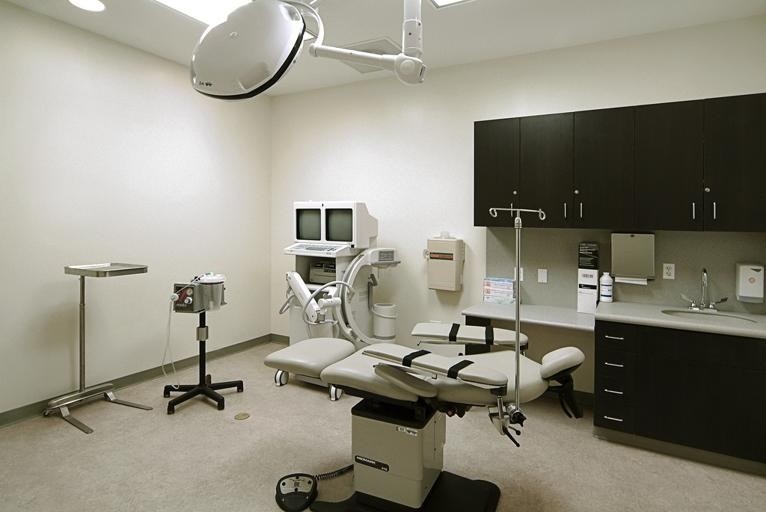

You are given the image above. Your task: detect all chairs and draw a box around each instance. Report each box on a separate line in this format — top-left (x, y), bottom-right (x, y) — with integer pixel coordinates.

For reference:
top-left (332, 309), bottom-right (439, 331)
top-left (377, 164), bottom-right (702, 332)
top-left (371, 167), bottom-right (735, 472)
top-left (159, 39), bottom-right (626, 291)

top-left (264, 336), bottom-right (588, 512)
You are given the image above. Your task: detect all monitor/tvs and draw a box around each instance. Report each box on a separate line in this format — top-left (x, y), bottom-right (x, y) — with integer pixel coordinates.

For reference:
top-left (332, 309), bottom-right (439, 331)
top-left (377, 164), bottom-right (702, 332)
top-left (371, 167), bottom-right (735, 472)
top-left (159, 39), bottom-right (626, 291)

top-left (292, 201), bottom-right (378, 249)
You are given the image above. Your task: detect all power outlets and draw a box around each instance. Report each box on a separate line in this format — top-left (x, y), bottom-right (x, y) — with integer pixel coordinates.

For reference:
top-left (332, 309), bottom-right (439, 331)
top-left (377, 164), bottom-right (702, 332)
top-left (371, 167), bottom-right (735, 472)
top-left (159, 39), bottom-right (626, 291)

top-left (184, 0), bottom-right (425, 100)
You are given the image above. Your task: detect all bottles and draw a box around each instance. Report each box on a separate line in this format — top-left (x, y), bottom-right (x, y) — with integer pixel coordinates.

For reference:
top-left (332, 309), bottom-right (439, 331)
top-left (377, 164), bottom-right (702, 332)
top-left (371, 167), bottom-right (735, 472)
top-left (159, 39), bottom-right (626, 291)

top-left (599, 272), bottom-right (614, 302)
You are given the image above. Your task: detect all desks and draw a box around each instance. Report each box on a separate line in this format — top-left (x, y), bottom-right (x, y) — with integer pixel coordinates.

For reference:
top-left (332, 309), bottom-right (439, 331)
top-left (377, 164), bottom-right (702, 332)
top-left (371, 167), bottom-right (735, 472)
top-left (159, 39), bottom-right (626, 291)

top-left (44, 260), bottom-right (150, 438)
top-left (460, 300), bottom-right (594, 354)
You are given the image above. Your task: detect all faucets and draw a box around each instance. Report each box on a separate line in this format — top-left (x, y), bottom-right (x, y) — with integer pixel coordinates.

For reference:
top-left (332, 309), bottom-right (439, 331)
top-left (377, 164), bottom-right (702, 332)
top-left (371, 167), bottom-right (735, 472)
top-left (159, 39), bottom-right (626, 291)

top-left (680, 270), bottom-right (729, 315)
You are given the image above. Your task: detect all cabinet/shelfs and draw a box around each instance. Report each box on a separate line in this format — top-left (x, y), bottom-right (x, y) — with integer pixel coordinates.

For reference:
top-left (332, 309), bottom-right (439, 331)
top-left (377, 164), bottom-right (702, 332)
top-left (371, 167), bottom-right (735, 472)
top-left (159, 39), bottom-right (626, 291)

top-left (519, 107), bottom-right (634, 232)
top-left (636, 93), bottom-right (766, 232)
top-left (596, 321), bottom-right (766, 464)
top-left (473, 118), bottom-right (519, 228)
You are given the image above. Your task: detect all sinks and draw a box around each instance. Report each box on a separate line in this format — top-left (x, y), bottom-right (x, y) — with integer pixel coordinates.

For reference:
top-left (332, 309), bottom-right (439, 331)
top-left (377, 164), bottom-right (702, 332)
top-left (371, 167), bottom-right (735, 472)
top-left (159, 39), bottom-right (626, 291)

top-left (657, 307), bottom-right (760, 329)
